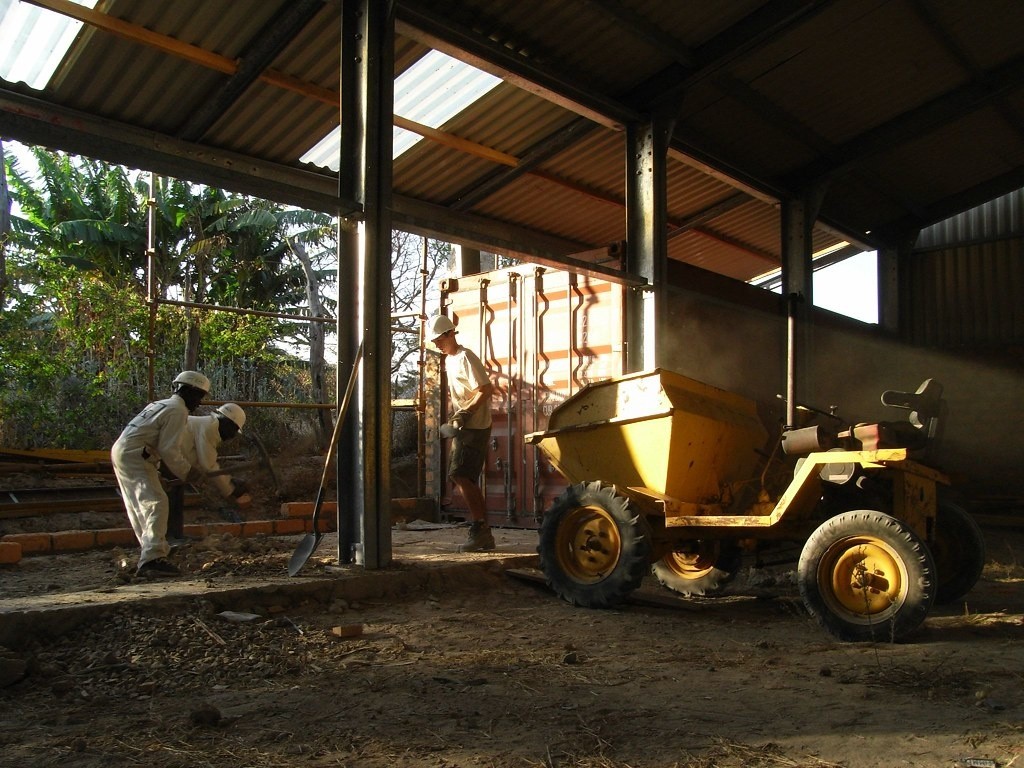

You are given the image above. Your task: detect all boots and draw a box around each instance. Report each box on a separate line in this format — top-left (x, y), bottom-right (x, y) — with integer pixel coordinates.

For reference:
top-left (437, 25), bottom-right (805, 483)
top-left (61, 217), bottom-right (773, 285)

top-left (457, 520), bottom-right (495, 551)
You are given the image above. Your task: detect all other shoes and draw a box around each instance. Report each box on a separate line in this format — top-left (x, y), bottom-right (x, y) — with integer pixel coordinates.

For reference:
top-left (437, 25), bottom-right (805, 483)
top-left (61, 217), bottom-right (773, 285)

top-left (167, 536), bottom-right (192, 544)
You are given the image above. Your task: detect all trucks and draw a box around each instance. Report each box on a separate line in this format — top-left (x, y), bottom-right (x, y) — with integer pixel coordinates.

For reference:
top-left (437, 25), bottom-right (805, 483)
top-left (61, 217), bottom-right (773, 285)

top-left (525, 290), bottom-right (986, 642)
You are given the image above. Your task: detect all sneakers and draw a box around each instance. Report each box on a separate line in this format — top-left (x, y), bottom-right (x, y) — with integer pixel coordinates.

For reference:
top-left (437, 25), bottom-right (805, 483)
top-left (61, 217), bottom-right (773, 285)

top-left (167, 545), bottom-right (178, 556)
top-left (136, 556), bottom-right (180, 575)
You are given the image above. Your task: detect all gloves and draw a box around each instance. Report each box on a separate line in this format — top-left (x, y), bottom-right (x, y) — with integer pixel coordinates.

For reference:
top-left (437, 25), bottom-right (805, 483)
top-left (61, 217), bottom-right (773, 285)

top-left (185, 465), bottom-right (200, 482)
top-left (449, 409), bottom-right (472, 432)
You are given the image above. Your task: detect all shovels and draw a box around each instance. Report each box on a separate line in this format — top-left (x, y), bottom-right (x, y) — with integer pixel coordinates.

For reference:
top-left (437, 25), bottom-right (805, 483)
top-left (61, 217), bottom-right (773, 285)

top-left (286, 340), bottom-right (364, 577)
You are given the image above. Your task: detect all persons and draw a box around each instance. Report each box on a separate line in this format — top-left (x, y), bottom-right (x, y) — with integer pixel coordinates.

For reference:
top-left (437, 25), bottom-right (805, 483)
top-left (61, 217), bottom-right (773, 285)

top-left (110, 371), bottom-right (210, 581)
top-left (157, 403), bottom-right (252, 552)
top-left (423, 315), bottom-right (496, 553)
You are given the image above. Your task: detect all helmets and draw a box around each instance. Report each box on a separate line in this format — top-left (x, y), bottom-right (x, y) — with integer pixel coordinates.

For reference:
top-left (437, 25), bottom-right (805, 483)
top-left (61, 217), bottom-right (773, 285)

top-left (216, 402), bottom-right (246, 433)
top-left (173, 370), bottom-right (209, 393)
top-left (425, 315), bottom-right (455, 341)
